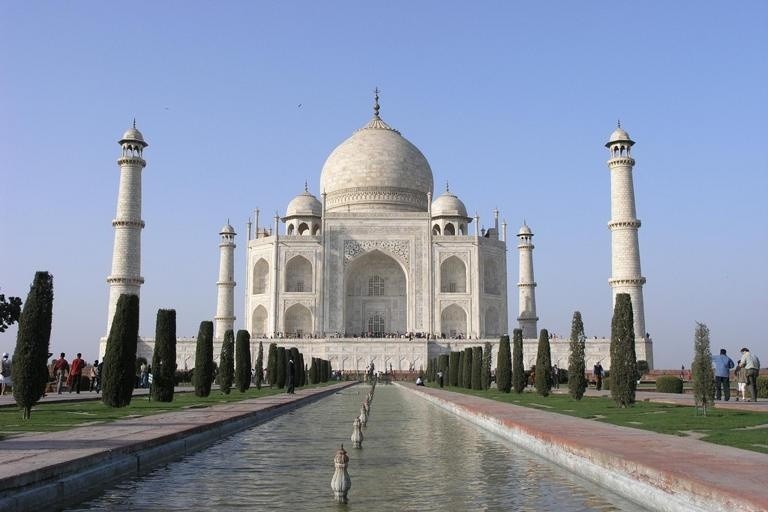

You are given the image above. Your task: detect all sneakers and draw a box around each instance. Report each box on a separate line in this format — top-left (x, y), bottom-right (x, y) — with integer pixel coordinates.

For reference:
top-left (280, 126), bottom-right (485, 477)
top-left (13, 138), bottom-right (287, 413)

top-left (714, 398), bottom-right (757, 403)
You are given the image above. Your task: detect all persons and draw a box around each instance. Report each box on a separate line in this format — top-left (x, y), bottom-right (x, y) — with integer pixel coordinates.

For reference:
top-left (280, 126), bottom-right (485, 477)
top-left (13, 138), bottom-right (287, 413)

top-left (134, 362), bottom-right (152, 388)
top-left (251, 367), bottom-right (268, 384)
top-left (681, 365), bottom-right (691, 380)
top-left (592, 362), bottom-right (603, 390)
top-left (43, 352), bottom-right (102, 396)
top-left (524, 370), bottom-right (530, 387)
top-left (263, 331), bottom-right (465, 339)
top-left (365, 365), bottom-right (382, 380)
top-left (552, 364), bottom-right (560, 389)
top-left (0, 353), bottom-right (13, 395)
top-left (738, 347), bottom-right (760, 402)
top-left (531, 371), bottom-right (537, 387)
top-left (330, 368), bottom-right (345, 376)
top-left (416, 376), bottom-right (424, 386)
top-left (734, 360), bottom-right (748, 401)
top-left (436, 369), bottom-right (443, 388)
top-left (711, 349), bottom-right (734, 401)
